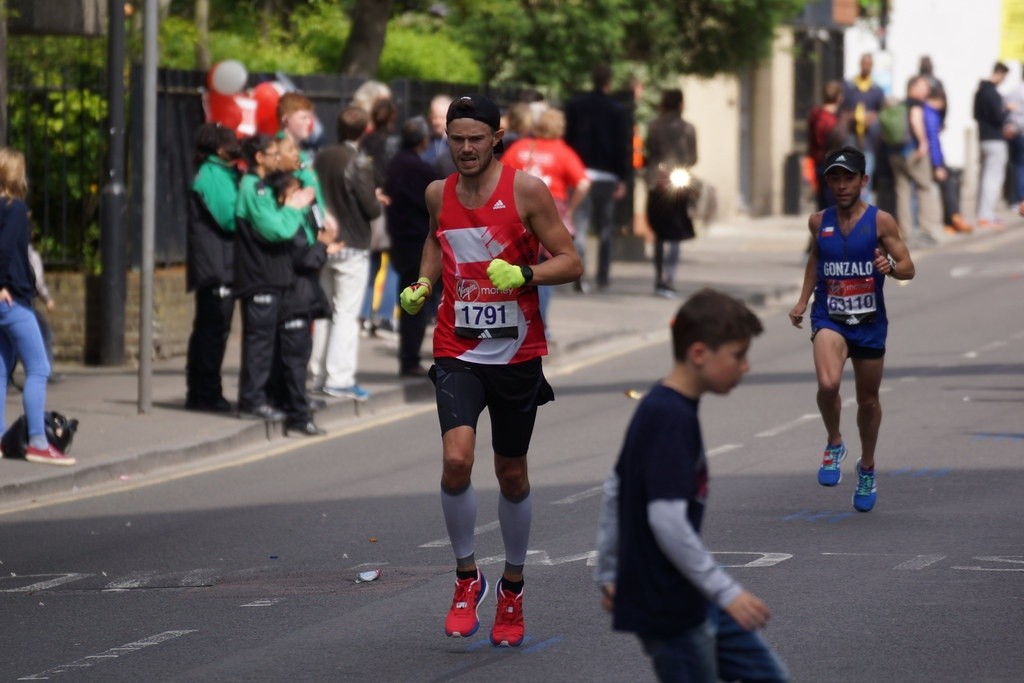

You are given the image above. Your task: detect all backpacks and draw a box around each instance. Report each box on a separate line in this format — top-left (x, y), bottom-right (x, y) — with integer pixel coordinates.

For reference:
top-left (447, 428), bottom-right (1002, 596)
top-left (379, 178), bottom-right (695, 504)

top-left (878, 102), bottom-right (916, 150)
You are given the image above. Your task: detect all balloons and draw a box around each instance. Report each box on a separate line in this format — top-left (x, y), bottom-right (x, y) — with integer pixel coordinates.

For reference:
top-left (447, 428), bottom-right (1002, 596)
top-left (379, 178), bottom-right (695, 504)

top-left (207, 59), bottom-right (322, 144)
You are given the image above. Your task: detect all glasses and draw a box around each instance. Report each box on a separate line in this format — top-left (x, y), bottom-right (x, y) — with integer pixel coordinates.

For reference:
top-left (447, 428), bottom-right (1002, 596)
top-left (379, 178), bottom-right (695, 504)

top-left (259, 150), bottom-right (279, 158)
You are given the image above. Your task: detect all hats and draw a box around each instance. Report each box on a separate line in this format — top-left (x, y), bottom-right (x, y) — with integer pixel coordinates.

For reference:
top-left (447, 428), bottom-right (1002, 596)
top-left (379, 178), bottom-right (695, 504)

top-left (446, 95), bottom-right (504, 154)
top-left (822, 147), bottom-right (867, 176)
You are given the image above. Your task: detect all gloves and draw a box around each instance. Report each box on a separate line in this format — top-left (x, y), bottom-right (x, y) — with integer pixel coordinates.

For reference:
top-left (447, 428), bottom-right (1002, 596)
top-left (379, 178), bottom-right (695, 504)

top-left (485, 258), bottom-right (527, 290)
top-left (400, 277), bottom-right (432, 315)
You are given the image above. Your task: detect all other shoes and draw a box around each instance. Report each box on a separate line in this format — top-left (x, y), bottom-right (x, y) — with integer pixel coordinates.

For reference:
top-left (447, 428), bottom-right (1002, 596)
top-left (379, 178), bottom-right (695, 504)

top-left (976, 218), bottom-right (1003, 230)
top-left (653, 282), bottom-right (677, 295)
top-left (186, 397), bottom-right (234, 413)
top-left (945, 215), bottom-right (970, 234)
top-left (398, 366), bottom-right (428, 379)
top-left (287, 420), bottom-right (326, 436)
top-left (238, 405), bottom-right (285, 421)
top-left (308, 398), bottom-right (326, 409)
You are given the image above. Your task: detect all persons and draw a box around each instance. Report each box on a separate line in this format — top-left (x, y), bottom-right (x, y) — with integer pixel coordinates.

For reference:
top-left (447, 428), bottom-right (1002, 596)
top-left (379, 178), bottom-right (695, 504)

top-left (173, 60), bottom-right (705, 436)
top-left (789, 148), bottom-right (915, 513)
top-left (807, 53), bottom-right (1024, 243)
top-left (400, 94), bottom-right (584, 647)
top-left (592, 289), bottom-right (790, 683)
top-left (0, 146), bottom-right (76, 466)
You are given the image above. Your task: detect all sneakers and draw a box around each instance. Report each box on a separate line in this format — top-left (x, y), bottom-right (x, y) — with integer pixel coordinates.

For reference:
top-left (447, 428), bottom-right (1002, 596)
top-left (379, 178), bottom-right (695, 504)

top-left (322, 382), bottom-right (371, 402)
top-left (491, 577), bottom-right (525, 647)
top-left (852, 457), bottom-right (877, 511)
top-left (445, 566), bottom-right (488, 639)
top-left (817, 441), bottom-right (848, 488)
top-left (24, 445), bottom-right (77, 466)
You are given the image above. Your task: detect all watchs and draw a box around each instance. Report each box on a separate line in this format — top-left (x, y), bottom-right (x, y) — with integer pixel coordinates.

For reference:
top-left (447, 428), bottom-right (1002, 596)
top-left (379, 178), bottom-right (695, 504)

top-left (519, 265), bottom-right (533, 287)
top-left (887, 258), bottom-right (896, 275)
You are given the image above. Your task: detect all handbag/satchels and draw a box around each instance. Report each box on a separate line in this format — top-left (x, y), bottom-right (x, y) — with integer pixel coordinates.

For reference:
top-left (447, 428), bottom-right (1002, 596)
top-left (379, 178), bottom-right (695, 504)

top-left (1, 411), bottom-right (79, 456)
